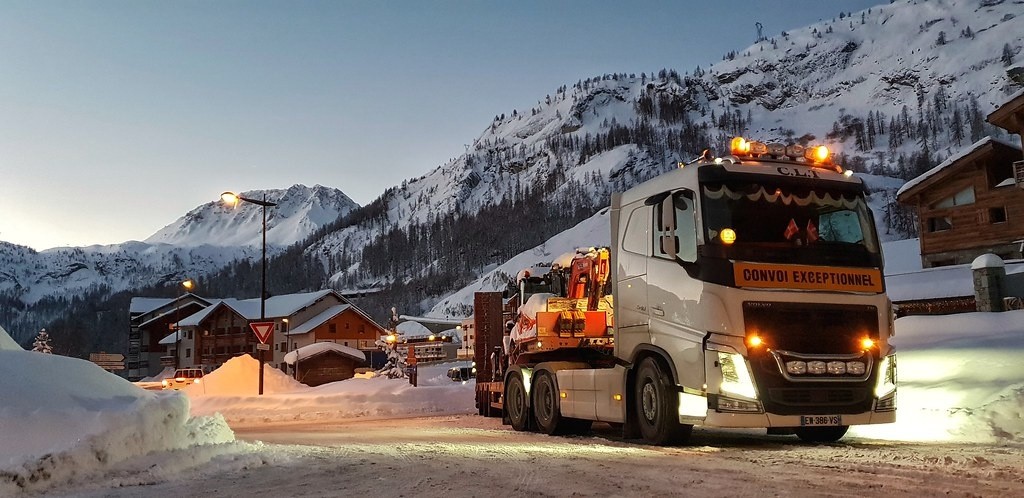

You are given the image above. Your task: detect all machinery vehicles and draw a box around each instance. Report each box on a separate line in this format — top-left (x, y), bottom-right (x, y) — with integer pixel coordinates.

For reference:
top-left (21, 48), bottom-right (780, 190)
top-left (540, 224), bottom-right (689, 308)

top-left (503, 251), bottom-right (614, 367)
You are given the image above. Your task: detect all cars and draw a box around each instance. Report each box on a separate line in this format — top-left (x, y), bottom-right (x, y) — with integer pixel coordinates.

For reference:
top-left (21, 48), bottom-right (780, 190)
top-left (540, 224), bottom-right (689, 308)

top-left (161, 369), bottom-right (204, 391)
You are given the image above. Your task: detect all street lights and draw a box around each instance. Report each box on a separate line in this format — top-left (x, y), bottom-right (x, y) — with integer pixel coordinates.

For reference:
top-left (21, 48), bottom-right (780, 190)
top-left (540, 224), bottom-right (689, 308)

top-left (220, 192), bottom-right (266, 395)
top-left (430, 336), bottom-right (438, 366)
top-left (175, 281), bottom-right (192, 370)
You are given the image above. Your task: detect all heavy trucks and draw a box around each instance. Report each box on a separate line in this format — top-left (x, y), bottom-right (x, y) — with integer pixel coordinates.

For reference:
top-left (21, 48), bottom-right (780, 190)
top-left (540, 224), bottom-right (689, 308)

top-left (473, 136), bottom-right (899, 443)
